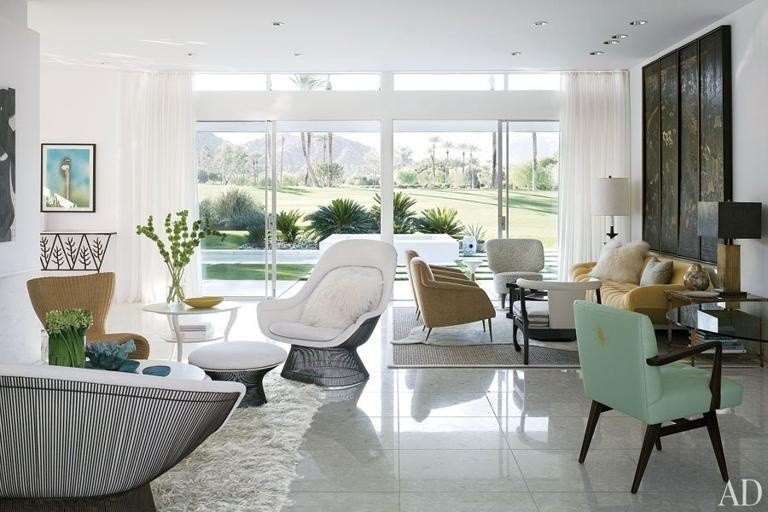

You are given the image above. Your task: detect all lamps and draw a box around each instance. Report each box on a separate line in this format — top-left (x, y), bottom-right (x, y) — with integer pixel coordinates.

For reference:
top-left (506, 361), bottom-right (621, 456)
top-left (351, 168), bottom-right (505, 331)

top-left (594, 178), bottom-right (626, 245)
top-left (698, 201), bottom-right (763, 298)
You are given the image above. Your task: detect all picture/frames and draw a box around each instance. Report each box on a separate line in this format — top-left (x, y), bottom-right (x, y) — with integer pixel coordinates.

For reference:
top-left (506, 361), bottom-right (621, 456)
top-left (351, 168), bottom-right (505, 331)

top-left (0, 86), bottom-right (17, 243)
top-left (40, 143), bottom-right (97, 214)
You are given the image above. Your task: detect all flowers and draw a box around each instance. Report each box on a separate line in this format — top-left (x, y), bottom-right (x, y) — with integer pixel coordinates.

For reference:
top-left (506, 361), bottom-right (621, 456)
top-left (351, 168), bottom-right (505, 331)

top-left (46, 308), bottom-right (94, 368)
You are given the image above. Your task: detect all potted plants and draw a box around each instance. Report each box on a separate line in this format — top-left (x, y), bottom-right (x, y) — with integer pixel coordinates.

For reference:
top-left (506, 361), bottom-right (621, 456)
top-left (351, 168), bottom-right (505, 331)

top-left (137, 210), bottom-right (227, 303)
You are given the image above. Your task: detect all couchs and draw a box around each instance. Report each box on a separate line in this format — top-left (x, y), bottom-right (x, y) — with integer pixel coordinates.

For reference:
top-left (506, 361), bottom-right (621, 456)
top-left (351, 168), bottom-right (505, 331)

top-left (573, 255), bottom-right (716, 330)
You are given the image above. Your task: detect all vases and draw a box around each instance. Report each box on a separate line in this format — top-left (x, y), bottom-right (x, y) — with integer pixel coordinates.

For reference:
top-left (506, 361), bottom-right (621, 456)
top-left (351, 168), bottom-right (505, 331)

top-left (49, 335), bottom-right (86, 368)
top-left (683, 263), bottom-right (709, 291)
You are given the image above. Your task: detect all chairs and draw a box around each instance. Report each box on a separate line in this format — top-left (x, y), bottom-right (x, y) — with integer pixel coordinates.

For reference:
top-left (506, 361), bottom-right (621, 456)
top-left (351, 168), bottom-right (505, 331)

top-left (573, 301), bottom-right (741, 495)
top-left (487, 239), bottom-right (544, 308)
top-left (406, 250), bottom-right (468, 320)
top-left (512, 278), bottom-right (602, 365)
top-left (411, 258), bottom-right (496, 344)
top-left (29, 273), bottom-right (151, 362)
top-left (257, 240), bottom-right (399, 387)
top-left (0, 365), bottom-right (246, 512)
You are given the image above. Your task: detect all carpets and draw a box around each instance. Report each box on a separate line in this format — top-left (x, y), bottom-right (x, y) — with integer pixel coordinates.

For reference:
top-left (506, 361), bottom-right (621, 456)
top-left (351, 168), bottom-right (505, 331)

top-left (389, 298), bottom-right (766, 369)
top-left (1, 366), bottom-right (325, 512)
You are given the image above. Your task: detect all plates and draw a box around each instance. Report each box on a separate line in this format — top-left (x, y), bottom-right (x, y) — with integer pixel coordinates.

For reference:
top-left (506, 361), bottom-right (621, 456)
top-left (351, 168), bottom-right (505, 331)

top-left (185, 297), bottom-right (225, 308)
top-left (687, 291), bottom-right (718, 298)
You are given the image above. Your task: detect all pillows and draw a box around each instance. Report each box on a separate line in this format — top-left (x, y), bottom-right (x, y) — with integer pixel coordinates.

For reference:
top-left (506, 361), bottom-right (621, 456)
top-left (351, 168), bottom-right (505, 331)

top-left (588, 239), bottom-right (649, 285)
top-left (639, 257), bottom-right (674, 286)
